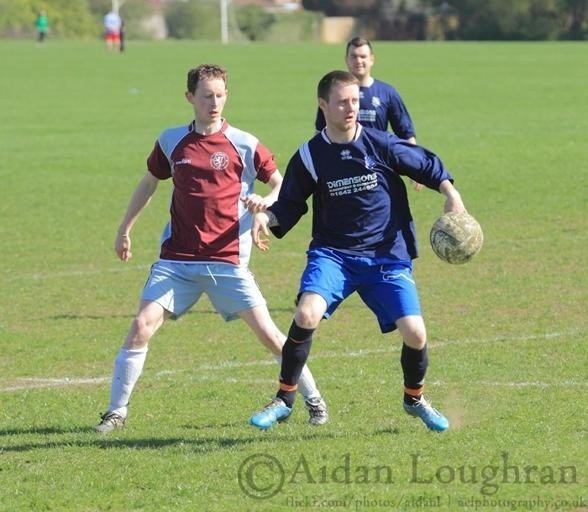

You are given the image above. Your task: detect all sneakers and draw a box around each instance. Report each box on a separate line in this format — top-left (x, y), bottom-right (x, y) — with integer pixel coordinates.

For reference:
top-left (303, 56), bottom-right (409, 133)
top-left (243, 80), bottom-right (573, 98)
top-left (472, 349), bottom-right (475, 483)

top-left (305, 396), bottom-right (329, 426)
top-left (403, 394), bottom-right (450, 431)
top-left (93, 411), bottom-right (126, 433)
top-left (250, 396), bottom-right (293, 428)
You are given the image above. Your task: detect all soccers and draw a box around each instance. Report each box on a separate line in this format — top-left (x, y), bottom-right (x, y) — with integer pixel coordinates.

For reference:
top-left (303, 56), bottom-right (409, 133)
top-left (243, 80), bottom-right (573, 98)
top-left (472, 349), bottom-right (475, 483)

top-left (430, 211), bottom-right (483, 267)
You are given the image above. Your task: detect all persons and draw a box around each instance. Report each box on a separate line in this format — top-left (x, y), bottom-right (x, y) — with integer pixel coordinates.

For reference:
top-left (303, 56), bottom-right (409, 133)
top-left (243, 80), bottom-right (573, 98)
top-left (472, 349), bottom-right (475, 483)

top-left (31, 10), bottom-right (49, 48)
top-left (313, 37), bottom-right (422, 194)
top-left (248, 70), bottom-right (468, 432)
top-left (92, 65), bottom-right (329, 435)
top-left (101, 10), bottom-right (127, 55)
